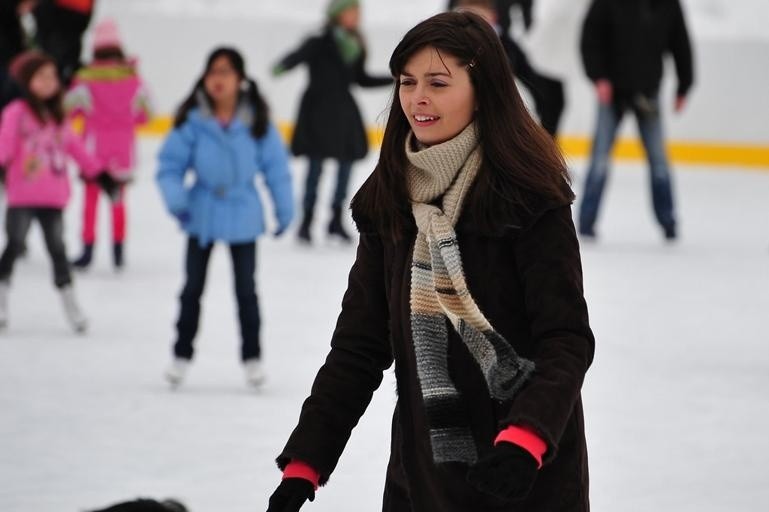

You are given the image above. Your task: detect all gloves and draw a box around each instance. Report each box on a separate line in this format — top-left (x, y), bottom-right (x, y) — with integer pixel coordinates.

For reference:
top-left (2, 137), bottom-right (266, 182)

top-left (266, 478), bottom-right (314, 512)
top-left (464, 442), bottom-right (539, 500)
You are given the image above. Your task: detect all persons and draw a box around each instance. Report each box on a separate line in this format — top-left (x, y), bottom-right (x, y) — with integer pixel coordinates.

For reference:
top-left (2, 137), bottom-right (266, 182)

top-left (0, 47), bottom-right (133, 339)
top-left (577, 1), bottom-right (694, 242)
top-left (1, 0), bottom-right (62, 108)
top-left (445, 1), bottom-right (566, 145)
top-left (67, 16), bottom-right (149, 270)
top-left (267, 0), bottom-right (393, 245)
top-left (263, 10), bottom-right (599, 512)
top-left (154, 43), bottom-right (297, 388)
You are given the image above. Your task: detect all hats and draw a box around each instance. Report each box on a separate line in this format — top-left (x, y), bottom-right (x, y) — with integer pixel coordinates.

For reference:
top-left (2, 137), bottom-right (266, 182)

top-left (93, 23), bottom-right (118, 48)
top-left (2, 49), bottom-right (50, 85)
top-left (329, 0), bottom-right (356, 20)
